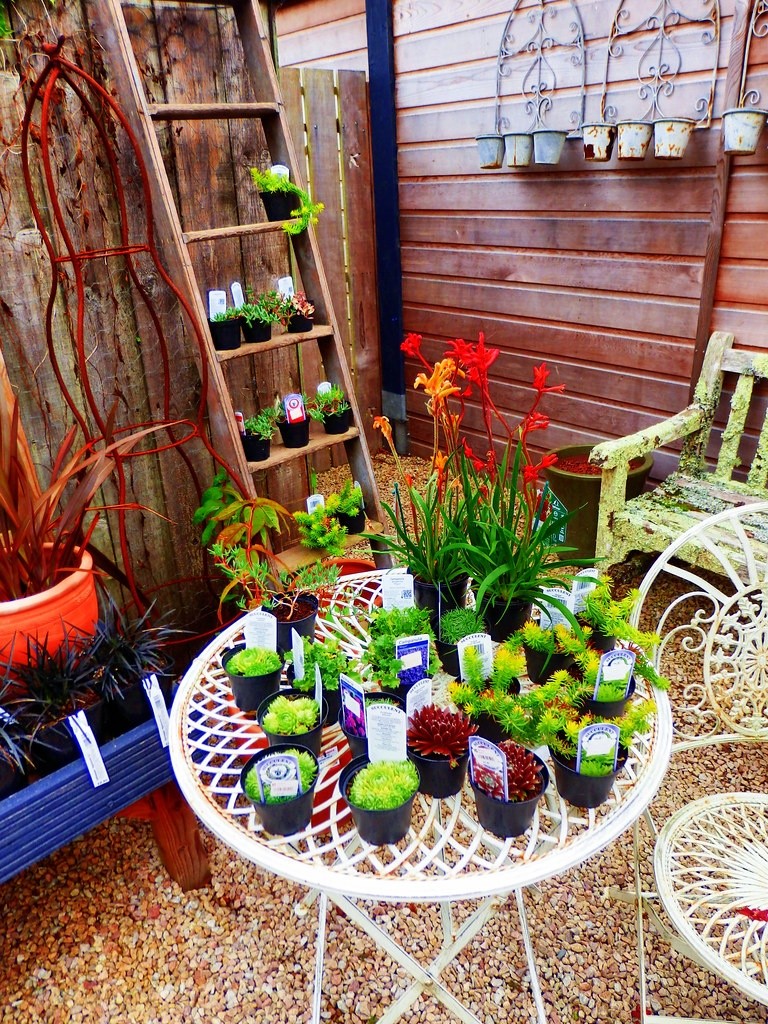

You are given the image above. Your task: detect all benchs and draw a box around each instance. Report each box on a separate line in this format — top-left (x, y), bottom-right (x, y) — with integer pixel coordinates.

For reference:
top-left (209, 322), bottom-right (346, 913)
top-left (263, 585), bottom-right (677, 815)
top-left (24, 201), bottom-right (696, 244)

top-left (590, 331), bottom-right (768, 590)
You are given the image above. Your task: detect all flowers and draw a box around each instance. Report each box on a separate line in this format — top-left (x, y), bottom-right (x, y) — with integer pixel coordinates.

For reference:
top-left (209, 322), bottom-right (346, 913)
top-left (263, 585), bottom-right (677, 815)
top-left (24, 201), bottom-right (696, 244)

top-left (369, 330), bottom-right (606, 677)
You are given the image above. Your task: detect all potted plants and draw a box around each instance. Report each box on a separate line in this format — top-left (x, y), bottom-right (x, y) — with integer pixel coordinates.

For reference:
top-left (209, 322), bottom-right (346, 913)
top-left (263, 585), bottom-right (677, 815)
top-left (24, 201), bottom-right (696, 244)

top-left (292, 479), bottom-right (365, 556)
top-left (208, 284), bottom-right (315, 350)
top-left (240, 384), bottom-right (351, 462)
top-left (208, 541), bottom-right (675, 846)
top-left (0, 346), bottom-right (199, 805)
top-left (249, 166), bottom-right (324, 236)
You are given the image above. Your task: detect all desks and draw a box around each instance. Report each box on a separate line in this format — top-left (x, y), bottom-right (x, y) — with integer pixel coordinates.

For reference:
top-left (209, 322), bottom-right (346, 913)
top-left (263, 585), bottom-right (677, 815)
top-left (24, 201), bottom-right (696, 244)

top-left (169, 567), bottom-right (673, 1024)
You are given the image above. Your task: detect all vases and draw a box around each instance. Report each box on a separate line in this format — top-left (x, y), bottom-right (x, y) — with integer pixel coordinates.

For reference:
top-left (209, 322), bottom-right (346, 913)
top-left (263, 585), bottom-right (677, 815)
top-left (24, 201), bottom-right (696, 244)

top-left (471, 578), bottom-right (533, 643)
top-left (542, 444), bottom-right (654, 561)
top-left (407, 565), bottom-right (471, 621)
top-left (474, 107), bottom-right (768, 169)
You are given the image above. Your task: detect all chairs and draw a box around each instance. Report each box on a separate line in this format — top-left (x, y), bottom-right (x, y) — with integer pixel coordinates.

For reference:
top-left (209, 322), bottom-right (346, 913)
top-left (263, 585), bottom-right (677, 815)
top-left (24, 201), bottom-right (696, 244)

top-left (608, 501), bottom-right (768, 1024)
